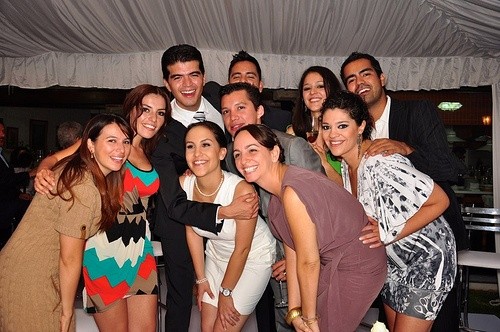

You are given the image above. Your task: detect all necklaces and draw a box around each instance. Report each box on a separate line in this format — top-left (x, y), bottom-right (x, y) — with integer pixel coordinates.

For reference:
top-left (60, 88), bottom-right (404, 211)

top-left (194, 175), bottom-right (224, 197)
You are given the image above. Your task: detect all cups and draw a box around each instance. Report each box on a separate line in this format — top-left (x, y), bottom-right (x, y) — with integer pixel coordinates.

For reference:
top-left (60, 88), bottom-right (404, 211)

top-left (305, 116), bottom-right (317, 143)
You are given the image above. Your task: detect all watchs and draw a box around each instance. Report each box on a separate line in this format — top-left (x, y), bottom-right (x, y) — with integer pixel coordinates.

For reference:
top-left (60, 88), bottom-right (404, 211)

top-left (219, 286), bottom-right (232, 297)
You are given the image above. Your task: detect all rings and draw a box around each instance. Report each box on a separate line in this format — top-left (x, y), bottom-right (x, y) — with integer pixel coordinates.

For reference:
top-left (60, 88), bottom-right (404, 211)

top-left (284, 270), bottom-right (287, 276)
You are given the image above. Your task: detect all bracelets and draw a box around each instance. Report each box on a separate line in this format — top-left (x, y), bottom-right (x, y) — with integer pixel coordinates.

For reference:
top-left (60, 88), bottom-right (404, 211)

top-left (196, 279), bottom-right (207, 284)
top-left (301, 315), bottom-right (320, 325)
top-left (286, 306), bottom-right (302, 322)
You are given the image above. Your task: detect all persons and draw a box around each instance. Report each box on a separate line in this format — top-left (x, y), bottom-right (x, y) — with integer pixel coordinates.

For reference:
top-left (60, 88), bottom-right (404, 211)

top-left (0, 43), bottom-right (469, 332)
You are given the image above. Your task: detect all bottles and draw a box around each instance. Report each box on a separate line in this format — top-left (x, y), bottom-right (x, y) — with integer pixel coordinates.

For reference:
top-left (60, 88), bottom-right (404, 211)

top-left (34, 150), bottom-right (43, 168)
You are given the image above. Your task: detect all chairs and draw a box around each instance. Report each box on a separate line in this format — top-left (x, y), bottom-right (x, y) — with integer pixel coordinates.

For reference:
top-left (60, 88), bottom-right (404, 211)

top-left (450, 204), bottom-right (500, 330)
